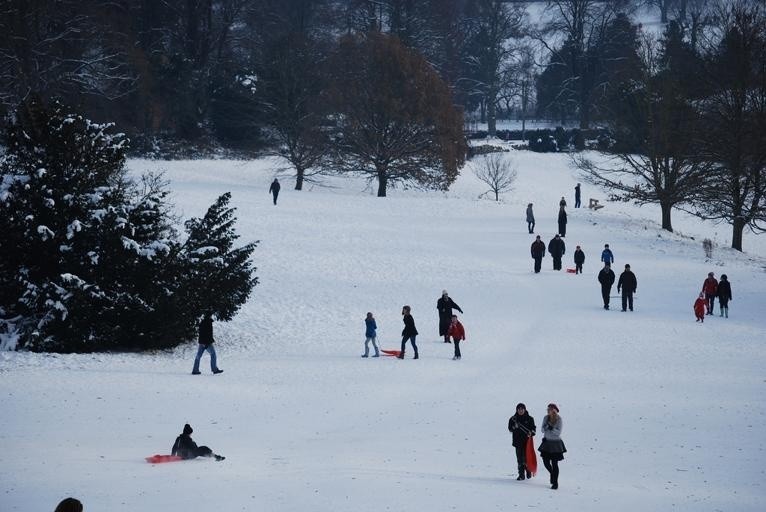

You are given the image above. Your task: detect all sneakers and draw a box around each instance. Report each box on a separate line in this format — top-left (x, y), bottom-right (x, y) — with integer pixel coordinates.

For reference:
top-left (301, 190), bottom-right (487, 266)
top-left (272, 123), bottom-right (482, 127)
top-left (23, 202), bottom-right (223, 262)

top-left (214, 368), bottom-right (223, 374)
top-left (550, 472), bottom-right (558, 489)
top-left (517, 472), bottom-right (531, 480)
top-left (192, 371), bottom-right (201, 374)
top-left (215, 456), bottom-right (225, 461)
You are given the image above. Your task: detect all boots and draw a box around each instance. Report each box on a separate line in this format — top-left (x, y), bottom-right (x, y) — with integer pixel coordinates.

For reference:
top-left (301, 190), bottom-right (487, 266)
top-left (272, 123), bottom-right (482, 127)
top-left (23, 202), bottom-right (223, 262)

top-left (362, 348), bottom-right (369, 357)
top-left (372, 347), bottom-right (379, 357)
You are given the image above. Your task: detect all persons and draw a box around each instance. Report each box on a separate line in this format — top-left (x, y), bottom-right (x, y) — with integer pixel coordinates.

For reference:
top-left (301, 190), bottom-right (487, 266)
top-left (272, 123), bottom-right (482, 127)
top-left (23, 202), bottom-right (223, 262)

top-left (446, 314), bottom-right (467, 361)
top-left (525, 202), bottom-right (536, 234)
top-left (436, 288), bottom-right (464, 345)
top-left (396, 304), bottom-right (420, 360)
top-left (617, 262), bottom-right (639, 313)
top-left (268, 177), bottom-right (281, 206)
top-left (600, 243), bottom-right (615, 269)
top-left (359, 311), bottom-right (381, 358)
top-left (701, 272), bottom-right (720, 315)
top-left (507, 402), bottom-right (537, 481)
top-left (53, 495), bottom-right (83, 512)
top-left (190, 309), bottom-right (224, 375)
top-left (598, 262), bottom-right (616, 311)
top-left (558, 206), bottom-right (568, 236)
top-left (714, 274), bottom-right (734, 319)
top-left (559, 196), bottom-right (567, 208)
top-left (547, 233), bottom-right (566, 272)
top-left (692, 292), bottom-right (709, 323)
top-left (574, 182), bottom-right (582, 209)
top-left (573, 245), bottom-right (586, 274)
top-left (530, 234), bottom-right (546, 275)
top-left (171, 423), bottom-right (226, 462)
top-left (537, 402), bottom-right (568, 490)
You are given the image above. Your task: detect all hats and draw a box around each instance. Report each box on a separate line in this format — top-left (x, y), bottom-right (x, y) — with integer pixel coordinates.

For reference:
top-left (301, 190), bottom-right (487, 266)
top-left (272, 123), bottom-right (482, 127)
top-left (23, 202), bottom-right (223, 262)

top-left (443, 290), bottom-right (448, 294)
top-left (548, 404), bottom-right (559, 413)
top-left (183, 424), bottom-right (193, 435)
